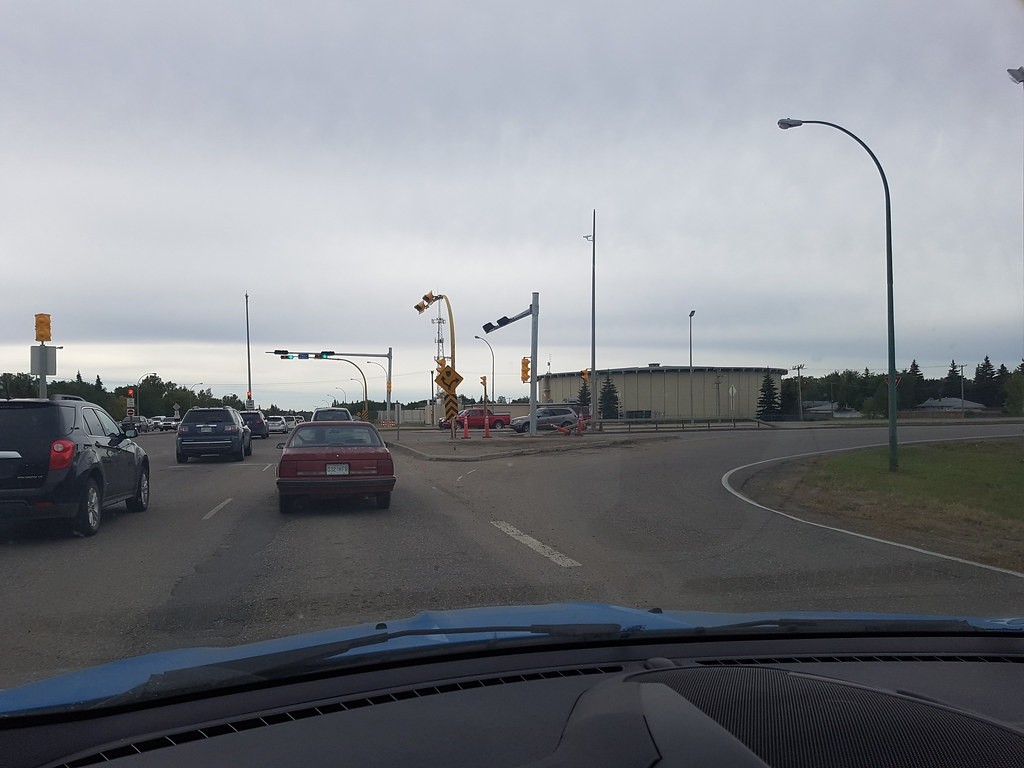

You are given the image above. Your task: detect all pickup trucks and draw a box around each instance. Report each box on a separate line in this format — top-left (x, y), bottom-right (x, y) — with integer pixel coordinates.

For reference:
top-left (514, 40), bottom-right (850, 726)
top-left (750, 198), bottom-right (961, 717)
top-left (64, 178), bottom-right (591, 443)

top-left (440, 408), bottom-right (511, 430)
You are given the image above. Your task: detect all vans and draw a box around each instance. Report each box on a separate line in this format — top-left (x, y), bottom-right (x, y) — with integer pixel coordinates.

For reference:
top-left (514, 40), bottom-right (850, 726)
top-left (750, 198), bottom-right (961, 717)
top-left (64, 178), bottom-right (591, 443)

top-left (311, 407), bottom-right (353, 445)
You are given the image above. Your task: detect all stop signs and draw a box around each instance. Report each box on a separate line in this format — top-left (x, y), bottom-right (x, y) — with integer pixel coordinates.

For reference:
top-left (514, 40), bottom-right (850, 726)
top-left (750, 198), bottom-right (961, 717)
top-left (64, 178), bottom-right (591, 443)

top-left (127, 410), bottom-right (134, 417)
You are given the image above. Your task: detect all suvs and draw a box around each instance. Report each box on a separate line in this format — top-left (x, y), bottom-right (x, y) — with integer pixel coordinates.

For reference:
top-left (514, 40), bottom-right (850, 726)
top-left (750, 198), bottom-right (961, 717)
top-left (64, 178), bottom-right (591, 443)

top-left (508, 408), bottom-right (579, 430)
top-left (0, 393), bottom-right (157, 537)
top-left (238, 410), bottom-right (270, 439)
top-left (174, 406), bottom-right (252, 463)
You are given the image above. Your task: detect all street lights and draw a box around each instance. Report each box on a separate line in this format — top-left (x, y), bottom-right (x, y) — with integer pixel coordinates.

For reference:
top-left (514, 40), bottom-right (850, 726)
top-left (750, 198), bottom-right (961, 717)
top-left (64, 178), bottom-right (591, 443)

top-left (327, 394), bottom-right (335, 406)
top-left (189, 383), bottom-right (203, 409)
top-left (321, 400), bottom-right (330, 409)
top-left (775, 118), bottom-right (903, 471)
top-left (335, 387), bottom-right (346, 405)
top-left (367, 362), bottom-right (388, 377)
top-left (137, 373), bottom-right (157, 415)
top-left (350, 378), bottom-right (365, 412)
top-left (688, 308), bottom-right (696, 428)
top-left (473, 335), bottom-right (495, 413)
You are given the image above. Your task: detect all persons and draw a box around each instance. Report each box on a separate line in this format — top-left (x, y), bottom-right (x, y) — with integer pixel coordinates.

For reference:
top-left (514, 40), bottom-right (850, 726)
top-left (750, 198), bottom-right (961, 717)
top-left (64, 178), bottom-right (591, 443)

top-left (314, 429), bottom-right (324, 441)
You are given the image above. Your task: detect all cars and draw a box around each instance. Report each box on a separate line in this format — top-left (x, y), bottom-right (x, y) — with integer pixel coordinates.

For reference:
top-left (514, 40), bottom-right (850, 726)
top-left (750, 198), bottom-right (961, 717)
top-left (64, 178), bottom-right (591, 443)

top-left (438, 409), bottom-right (466, 428)
top-left (119, 415), bottom-right (166, 433)
top-left (159, 417), bottom-right (180, 431)
top-left (284, 415), bottom-right (297, 430)
top-left (274, 421), bottom-right (397, 513)
top-left (295, 416), bottom-right (306, 426)
top-left (266, 415), bottom-right (288, 434)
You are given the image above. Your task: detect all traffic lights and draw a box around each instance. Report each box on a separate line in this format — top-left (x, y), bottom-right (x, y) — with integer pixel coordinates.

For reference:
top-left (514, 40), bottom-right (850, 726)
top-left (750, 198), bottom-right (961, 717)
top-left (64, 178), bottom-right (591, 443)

top-left (422, 289), bottom-right (433, 303)
top-left (580, 370), bottom-right (590, 383)
top-left (436, 359), bottom-right (446, 374)
top-left (315, 353), bottom-right (327, 358)
top-left (414, 301), bottom-right (426, 313)
top-left (386, 379), bottom-right (392, 392)
top-left (480, 376), bottom-right (486, 386)
top-left (247, 392), bottom-right (251, 401)
top-left (521, 359), bottom-right (530, 382)
top-left (280, 355), bottom-right (294, 359)
